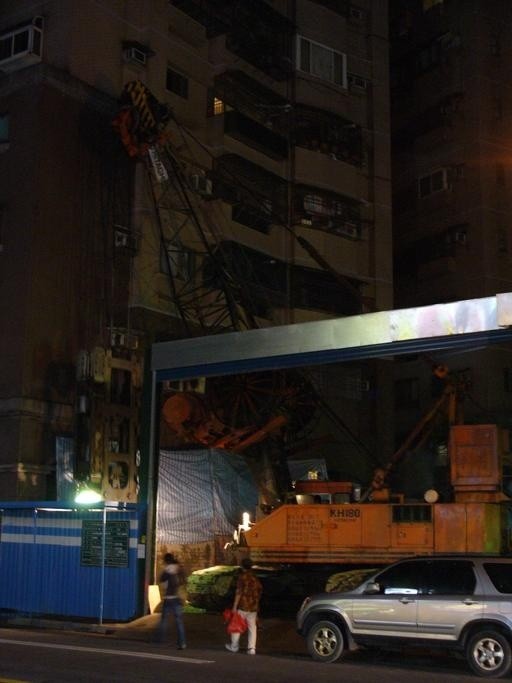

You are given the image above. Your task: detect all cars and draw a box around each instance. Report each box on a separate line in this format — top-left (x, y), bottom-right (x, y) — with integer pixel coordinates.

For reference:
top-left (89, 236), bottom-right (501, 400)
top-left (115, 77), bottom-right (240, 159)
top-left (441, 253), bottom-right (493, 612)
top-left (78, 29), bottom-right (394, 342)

top-left (294, 555), bottom-right (512, 679)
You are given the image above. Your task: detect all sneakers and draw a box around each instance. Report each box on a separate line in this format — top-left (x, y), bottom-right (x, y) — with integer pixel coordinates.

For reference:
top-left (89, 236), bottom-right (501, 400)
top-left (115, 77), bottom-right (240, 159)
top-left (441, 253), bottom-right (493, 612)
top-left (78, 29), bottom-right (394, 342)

top-left (226, 642), bottom-right (256, 655)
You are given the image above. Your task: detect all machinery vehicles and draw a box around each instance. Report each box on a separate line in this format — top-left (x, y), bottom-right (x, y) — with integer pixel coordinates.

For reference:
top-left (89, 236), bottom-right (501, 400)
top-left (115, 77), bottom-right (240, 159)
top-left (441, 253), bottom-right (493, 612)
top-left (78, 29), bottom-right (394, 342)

top-left (70, 79), bottom-right (512, 619)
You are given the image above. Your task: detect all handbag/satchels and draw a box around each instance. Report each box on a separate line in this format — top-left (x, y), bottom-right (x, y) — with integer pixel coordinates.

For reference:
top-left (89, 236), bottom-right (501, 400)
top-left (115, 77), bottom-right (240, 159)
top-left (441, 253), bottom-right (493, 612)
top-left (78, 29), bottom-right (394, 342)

top-left (176, 580), bottom-right (189, 607)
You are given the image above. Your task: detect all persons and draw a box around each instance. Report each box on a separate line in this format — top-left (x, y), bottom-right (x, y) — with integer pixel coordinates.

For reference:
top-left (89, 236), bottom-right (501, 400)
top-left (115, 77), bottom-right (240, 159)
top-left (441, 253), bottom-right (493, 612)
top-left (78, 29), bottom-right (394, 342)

top-left (221, 556), bottom-right (260, 657)
top-left (155, 552), bottom-right (187, 651)
